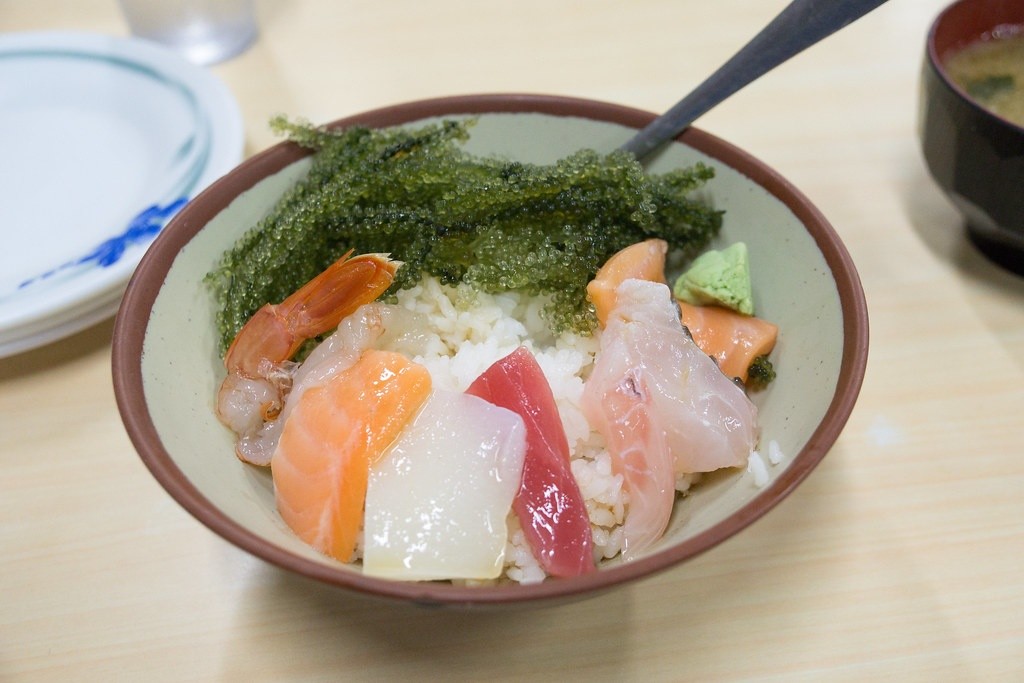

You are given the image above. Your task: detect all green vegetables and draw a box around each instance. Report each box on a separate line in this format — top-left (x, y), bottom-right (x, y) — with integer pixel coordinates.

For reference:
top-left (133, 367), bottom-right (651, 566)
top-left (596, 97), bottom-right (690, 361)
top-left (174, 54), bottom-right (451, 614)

top-left (953, 72), bottom-right (1017, 99)
top-left (210, 117), bottom-right (726, 333)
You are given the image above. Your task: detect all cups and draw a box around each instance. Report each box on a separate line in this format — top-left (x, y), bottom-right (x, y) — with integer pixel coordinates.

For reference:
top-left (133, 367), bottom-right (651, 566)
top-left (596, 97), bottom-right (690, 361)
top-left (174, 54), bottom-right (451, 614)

top-left (117, 0), bottom-right (259, 67)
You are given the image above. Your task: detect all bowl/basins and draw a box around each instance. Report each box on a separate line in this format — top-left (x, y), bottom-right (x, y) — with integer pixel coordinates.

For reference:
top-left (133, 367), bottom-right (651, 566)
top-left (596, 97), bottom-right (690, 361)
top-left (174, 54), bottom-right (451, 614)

top-left (111, 87), bottom-right (869, 615)
top-left (916, 0), bottom-right (1023, 275)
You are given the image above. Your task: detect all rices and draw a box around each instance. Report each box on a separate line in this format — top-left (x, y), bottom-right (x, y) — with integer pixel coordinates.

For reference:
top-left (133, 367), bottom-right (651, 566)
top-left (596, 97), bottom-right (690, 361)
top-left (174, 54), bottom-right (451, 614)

top-left (384, 284), bottom-right (700, 586)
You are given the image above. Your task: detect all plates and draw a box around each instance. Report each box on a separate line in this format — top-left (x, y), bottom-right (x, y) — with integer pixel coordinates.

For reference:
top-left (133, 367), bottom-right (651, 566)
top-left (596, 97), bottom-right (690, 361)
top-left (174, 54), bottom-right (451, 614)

top-left (0, 35), bottom-right (246, 361)
